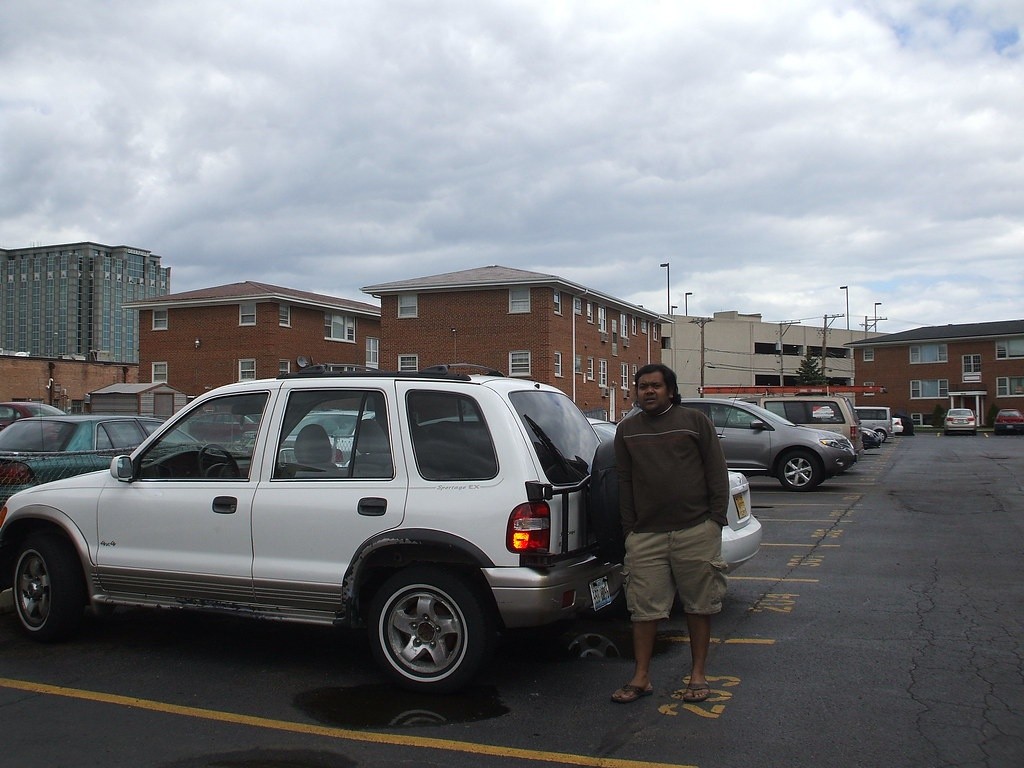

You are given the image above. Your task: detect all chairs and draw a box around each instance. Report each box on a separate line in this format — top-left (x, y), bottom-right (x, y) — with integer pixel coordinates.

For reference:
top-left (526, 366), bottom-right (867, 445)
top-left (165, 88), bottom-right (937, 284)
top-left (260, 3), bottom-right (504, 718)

top-left (347, 420), bottom-right (392, 477)
top-left (294, 424), bottom-right (338, 478)
top-left (712, 407), bottom-right (733, 427)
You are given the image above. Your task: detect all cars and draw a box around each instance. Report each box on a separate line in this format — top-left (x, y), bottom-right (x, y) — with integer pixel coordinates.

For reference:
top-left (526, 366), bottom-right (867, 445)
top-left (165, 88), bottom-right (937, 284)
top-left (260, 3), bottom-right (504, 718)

top-left (189, 413), bottom-right (260, 444)
top-left (402, 413), bottom-right (763, 612)
top-left (892, 418), bottom-right (904, 433)
top-left (0, 414), bottom-right (201, 510)
top-left (992, 408), bottom-right (1024, 435)
top-left (0, 402), bottom-right (70, 433)
top-left (279, 410), bottom-right (377, 470)
top-left (941, 408), bottom-right (978, 435)
top-left (861, 427), bottom-right (881, 450)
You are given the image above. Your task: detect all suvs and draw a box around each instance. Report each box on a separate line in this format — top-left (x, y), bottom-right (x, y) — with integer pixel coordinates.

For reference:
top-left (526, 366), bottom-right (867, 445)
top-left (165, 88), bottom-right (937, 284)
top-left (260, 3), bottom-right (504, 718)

top-left (616, 398), bottom-right (858, 492)
top-left (0, 362), bottom-right (625, 698)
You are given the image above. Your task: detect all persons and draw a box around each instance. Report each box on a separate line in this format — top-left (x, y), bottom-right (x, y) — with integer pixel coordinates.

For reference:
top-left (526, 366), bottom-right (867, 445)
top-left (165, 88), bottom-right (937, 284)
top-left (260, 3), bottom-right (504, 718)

top-left (611, 363), bottom-right (729, 704)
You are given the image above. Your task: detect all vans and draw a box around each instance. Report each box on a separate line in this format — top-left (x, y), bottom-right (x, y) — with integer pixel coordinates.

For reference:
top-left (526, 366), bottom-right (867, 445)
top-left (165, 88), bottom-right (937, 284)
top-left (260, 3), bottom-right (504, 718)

top-left (853, 407), bottom-right (892, 444)
top-left (736, 396), bottom-right (865, 459)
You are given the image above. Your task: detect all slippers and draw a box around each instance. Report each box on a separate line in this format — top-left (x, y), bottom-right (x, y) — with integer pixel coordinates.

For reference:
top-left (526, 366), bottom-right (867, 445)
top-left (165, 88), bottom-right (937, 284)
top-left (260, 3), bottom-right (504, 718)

top-left (611, 684), bottom-right (653, 704)
top-left (684, 680), bottom-right (709, 702)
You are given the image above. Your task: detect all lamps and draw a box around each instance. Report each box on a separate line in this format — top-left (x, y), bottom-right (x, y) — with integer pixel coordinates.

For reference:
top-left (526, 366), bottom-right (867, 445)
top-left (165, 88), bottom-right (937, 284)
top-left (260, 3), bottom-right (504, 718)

top-left (195, 337), bottom-right (200, 349)
